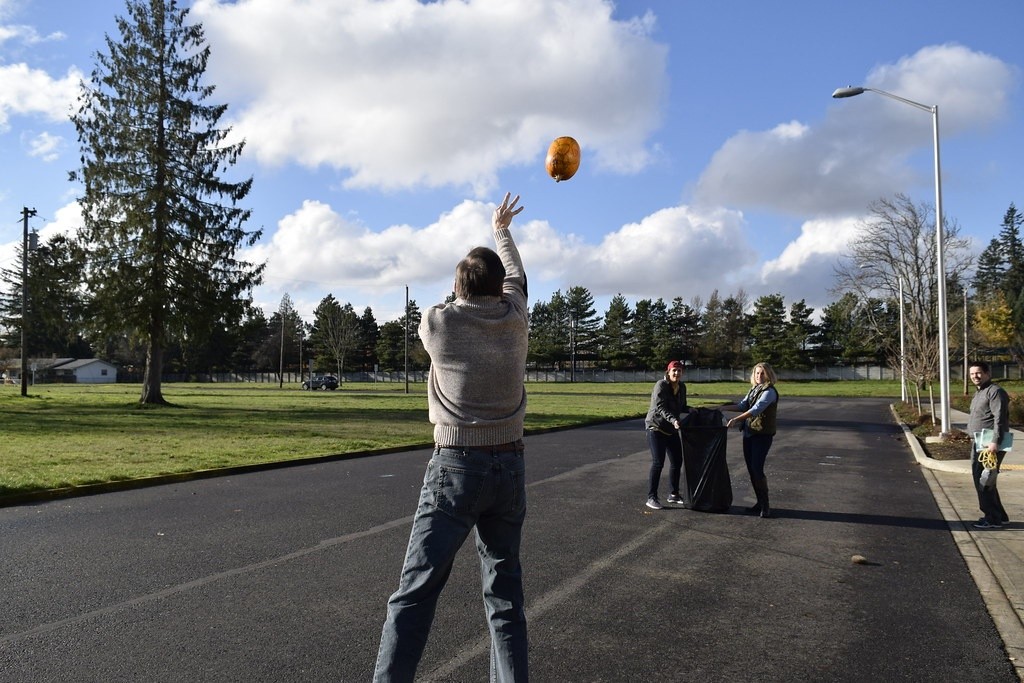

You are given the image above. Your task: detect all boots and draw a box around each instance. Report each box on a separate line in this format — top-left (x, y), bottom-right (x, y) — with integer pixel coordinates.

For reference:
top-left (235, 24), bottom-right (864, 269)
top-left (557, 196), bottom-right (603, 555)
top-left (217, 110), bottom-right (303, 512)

top-left (747, 502), bottom-right (760, 514)
top-left (753, 477), bottom-right (771, 517)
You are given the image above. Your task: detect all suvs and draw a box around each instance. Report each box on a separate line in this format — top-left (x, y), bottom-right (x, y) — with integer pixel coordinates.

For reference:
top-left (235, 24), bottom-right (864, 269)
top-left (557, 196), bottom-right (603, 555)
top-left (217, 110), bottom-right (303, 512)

top-left (302, 376), bottom-right (339, 390)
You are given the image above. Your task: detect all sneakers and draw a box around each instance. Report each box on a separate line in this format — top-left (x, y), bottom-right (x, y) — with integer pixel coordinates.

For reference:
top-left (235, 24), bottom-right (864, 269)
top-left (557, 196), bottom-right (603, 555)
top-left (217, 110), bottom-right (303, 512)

top-left (972, 522), bottom-right (1003, 528)
top-left (646, 498), bottom-right (662, 509)
top-left (979, 517), bottom-right (1010, 525)
top-left (668, 493), bottom-right (683, 504)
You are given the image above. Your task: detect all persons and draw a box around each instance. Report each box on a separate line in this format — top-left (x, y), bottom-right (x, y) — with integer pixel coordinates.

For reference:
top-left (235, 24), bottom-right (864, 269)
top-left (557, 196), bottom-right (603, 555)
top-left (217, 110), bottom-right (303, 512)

top-left (717, 363), bottom-right (779, 517)
top-left (372, 191), bottom-right (530, 683)
top-left (967, 362), bottom-right (1010, 529)
top-left (644, 360), bottom-right (687, 509)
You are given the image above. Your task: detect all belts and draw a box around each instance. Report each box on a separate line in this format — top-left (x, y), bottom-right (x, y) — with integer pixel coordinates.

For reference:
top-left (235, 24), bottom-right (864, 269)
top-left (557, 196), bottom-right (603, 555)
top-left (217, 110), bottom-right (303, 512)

top-left (439, 440), bottom-right (525, 452)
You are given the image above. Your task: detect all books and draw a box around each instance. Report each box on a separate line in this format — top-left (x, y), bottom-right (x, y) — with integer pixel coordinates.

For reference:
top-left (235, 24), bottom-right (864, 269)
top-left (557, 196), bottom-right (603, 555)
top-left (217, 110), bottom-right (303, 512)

top-left (974, 428), bottom-right (1014, 452)
